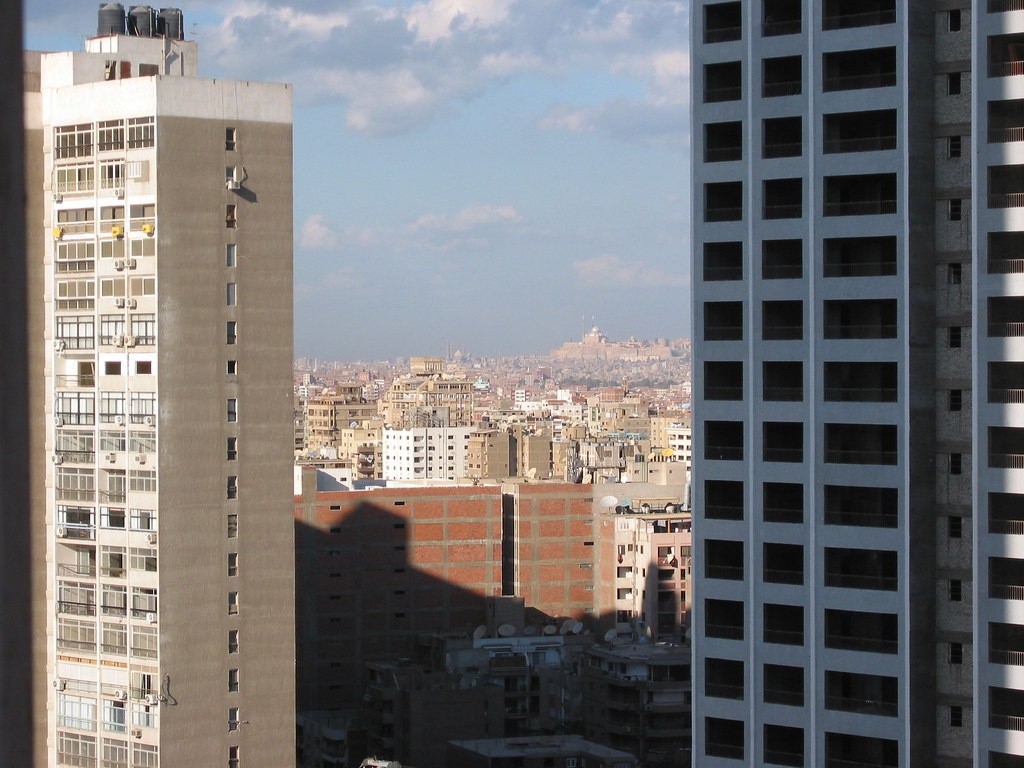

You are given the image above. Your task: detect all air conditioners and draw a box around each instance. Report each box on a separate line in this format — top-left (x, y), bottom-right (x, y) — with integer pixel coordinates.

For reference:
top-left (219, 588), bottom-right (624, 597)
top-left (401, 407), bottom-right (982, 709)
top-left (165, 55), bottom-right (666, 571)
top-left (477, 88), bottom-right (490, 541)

top-left (135, 455), bottom-right (146, 462)
top-left (57, 528), bottom-right (67, 536)
top-left (54, 418), bottom-right (63, 426)
top-left (145, 416), bottom-right (155, 426)
top-left (54, 193), bottom-right (61, 200)
top-left (124, 337), bottom-right (135, 346)
top-left (146, 534), bottom-right (156, 542)
top-left (105, 455), bottom-right (115, 461)
top-left (52, 455), bottom-right (62, 464)
top-left (53, 679), bottom-right (157, 738)
top-left (112, 260), bottom-right (123, 269)
top-left (112, 336), bottom-right (123, 345)
top-left (124, 259), bottom-right (135, 267)
top-left (114, 189), bottom-right (124, 197)
top-left (53, 340), bottom-right (63, 350)
top-left (115, 298), bottom-right (123, 306)
top-left (115, 416), bottom-right (124, 423)
top-left (127, 299), bottom-right (135, 307)
top-left (228, 182), bottom-right (240, 189)
top-left (146, 612), bottom-right (156, 622)
